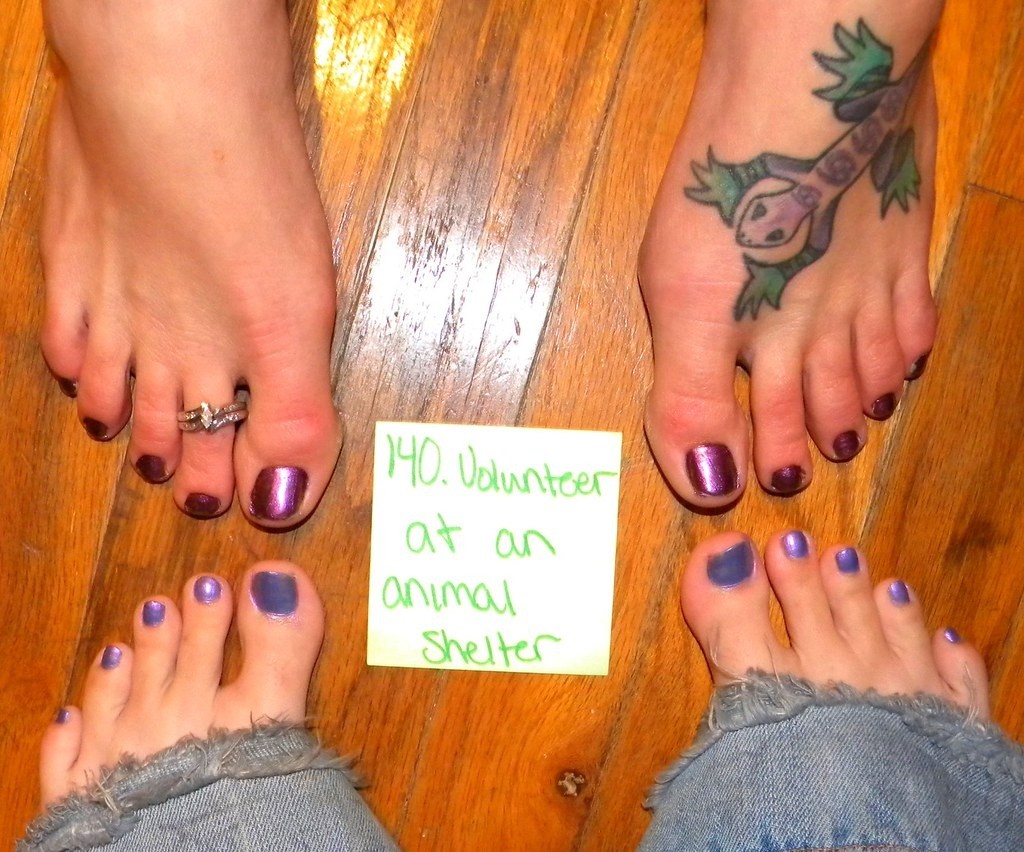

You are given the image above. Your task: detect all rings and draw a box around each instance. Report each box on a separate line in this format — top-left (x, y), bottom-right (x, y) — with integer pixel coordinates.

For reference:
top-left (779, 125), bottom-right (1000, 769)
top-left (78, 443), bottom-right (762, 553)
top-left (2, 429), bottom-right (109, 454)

top-left (177, 401), bottom-right (248, 434)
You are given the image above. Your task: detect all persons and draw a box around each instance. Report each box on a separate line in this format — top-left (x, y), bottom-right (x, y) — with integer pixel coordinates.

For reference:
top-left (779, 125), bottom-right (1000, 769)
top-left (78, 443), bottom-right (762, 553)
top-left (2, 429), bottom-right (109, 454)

top-left (16, 531), bottom-right (1024, 852)
top-left (39, 0), bottom-right (948, 531)
